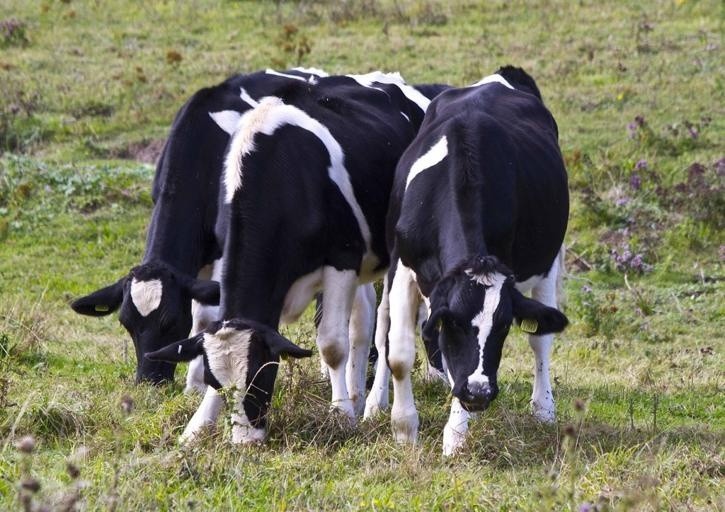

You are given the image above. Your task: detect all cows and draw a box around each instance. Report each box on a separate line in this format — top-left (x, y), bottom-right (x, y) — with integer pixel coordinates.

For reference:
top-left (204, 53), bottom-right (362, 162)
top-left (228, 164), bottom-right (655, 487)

top-left (70, 67), bottom-right (378, 395)
top-left (385, 65), bottom-right (570, 459)
top-left (146, 83), bottom-right (454, 450)
